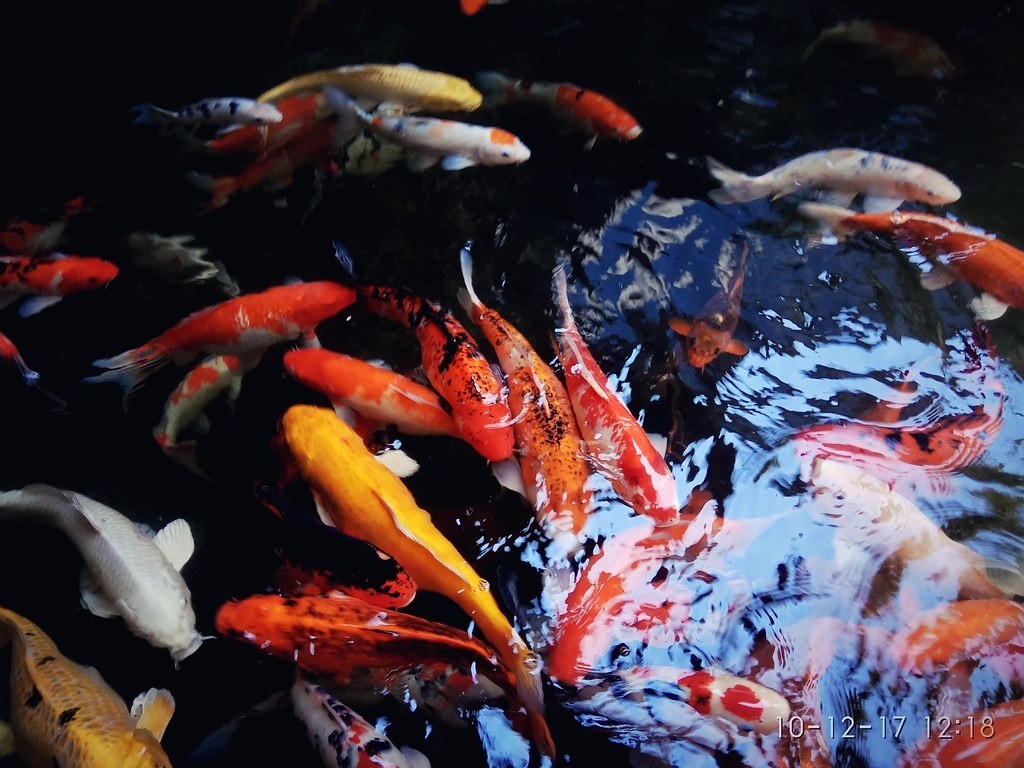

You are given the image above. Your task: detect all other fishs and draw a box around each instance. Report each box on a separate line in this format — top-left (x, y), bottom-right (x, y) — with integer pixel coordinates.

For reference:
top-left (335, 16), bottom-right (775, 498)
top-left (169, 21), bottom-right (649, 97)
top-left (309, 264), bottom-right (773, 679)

top-left (1, 0), bottom-right (1023, 768)
